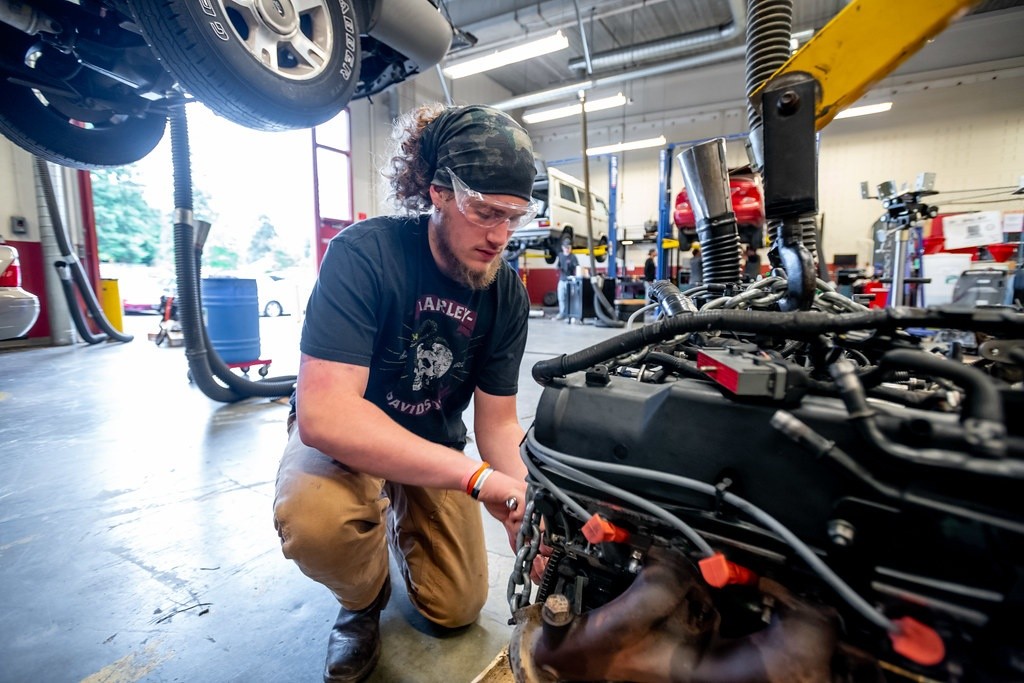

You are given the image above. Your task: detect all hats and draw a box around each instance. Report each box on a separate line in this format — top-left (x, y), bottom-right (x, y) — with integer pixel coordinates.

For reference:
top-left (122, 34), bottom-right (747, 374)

top-left (562, 238), bottom-right (572, 247)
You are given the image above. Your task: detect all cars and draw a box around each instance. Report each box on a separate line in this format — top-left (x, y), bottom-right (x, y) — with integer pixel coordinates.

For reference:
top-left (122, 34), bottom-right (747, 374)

top-left (1, 237), bottom-right (40, 341)
top-left (256, 267), bottom-right (318, 317)
top-left (0, 1), bottom-right (453, 172)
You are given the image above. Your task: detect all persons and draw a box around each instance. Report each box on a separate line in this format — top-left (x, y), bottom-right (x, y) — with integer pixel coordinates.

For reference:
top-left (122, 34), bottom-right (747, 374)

top-left (742, 246), bottom-right (761, 284)
top-left (689, 248), bottom-right (703, 286)
top-left (643, 248), bottom-right (658, 316)
top-left (551, 240), bottom-right (581, 322)
top-left (273, 103), bottom-right (555, 683)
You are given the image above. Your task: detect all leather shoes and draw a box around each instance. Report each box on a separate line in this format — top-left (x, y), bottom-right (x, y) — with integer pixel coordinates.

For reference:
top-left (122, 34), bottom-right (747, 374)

top-left (323, 575), bottom-right (392, 683)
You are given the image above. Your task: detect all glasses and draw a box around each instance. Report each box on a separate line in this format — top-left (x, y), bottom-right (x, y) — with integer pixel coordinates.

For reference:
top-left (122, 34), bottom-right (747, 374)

top-left (445, 166), bottom-right (538, 231)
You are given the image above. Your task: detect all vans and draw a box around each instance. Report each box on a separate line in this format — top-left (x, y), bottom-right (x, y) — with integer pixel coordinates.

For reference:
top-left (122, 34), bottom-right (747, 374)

top-left (504, 151), bottom-right (624, 264)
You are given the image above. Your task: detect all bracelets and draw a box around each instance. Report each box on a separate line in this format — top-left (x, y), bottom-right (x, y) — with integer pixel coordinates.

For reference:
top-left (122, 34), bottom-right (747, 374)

top-left (461, 461), bottom-right (495, 500)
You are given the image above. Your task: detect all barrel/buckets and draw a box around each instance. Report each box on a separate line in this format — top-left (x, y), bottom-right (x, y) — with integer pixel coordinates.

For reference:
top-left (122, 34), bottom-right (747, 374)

top-left (197, 276), bottom-right (261, 363)
